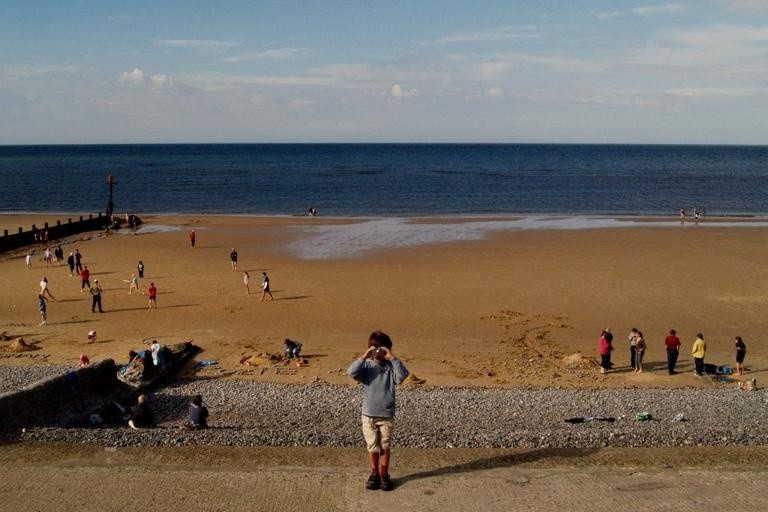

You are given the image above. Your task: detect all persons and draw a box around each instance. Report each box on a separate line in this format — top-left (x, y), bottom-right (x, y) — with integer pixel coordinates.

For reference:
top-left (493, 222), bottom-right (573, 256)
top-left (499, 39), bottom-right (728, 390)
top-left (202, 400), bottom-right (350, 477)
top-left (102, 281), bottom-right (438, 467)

top-left (128, 273), bottom-right (144, 296)
top-left (243, 271), bottom-right (250, 294)
top-left (89, 280), bottom-right (104, 313)
top-left (102, 211), bottom-right (137, 235)
top-left (309, 208), bottom-right (318, 219)
top-left (39, 276), bottom-right (56, 302)
top-left (348, 332), bottom-right (408, 490)
top-left (260, 272), bottom-right (274, 301)
top-left (78, 354), bottom-right (90, 367)
top-left (26, 229), bottom-right (83, 278)
top-left (284, 339), bottom-right (302, 359)
top-left (189, 230), bottom-right (196, 249)
top-left (694, 208), bottom-right (699, 225)
top-left (127, 395), bottom-right (157, 429)
top-left (599, 326), bottom-right (646, 373)
top-left (129, 350), bottom-right (138, 363)
top-left (230, 248), bottom-right (238, 272)
top-left (148, 282), bottom-right (157, 312)
top-left (691, 333), bottom-right (707, 376)
top-left (148, 339), bottom-right (166, 369)
top-left (664, 329), bottom-right (681, 375)
top-left (37, 294), bottom-right (48, 327)
top-left (680, 208), bottom-right (686, 221)
top-left (186, 394), bottom-right (210, 428)
top-left (136, 260), bottom-right (144, 280)
top-left (734, 336), bottom-right (746, 376)
top-left (88, 330), bottom-right (96, 344)
top-left (79, 264), bottom-right (92, 292)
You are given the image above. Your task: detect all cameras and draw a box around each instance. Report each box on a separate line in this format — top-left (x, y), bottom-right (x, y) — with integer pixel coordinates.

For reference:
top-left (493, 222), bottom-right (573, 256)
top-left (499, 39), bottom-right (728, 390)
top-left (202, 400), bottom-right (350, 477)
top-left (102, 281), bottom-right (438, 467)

top-left (372, 348), bottom-right (384, 355)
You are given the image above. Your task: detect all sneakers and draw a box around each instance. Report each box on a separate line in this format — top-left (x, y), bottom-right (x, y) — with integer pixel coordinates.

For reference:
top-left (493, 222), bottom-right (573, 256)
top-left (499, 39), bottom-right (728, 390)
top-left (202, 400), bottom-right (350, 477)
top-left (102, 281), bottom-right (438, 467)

top-left (366, 474), bottom-right (380, 489)
top-left (381, 475), bottom-right (393, 491)
